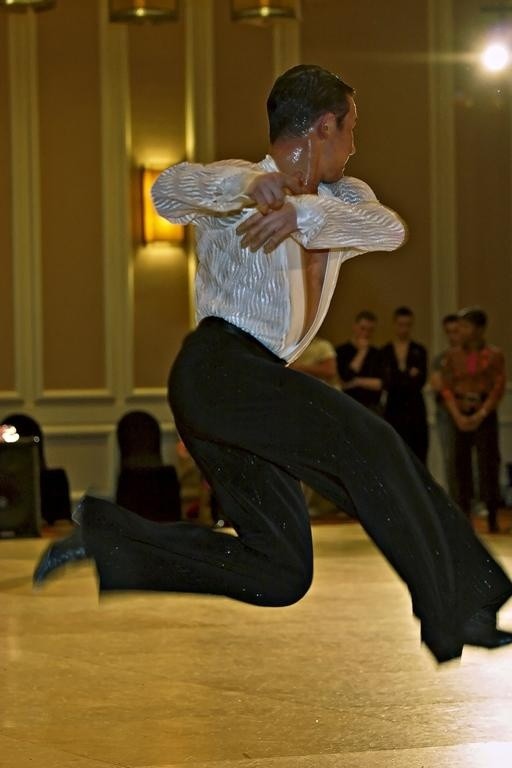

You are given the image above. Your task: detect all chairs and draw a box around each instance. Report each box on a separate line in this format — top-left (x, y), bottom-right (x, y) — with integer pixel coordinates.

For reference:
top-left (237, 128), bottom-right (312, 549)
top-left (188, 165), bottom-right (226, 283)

top-left (116, 411), bottom-right (182, 522)
top-left (1, 414), bottom-right (72, 525)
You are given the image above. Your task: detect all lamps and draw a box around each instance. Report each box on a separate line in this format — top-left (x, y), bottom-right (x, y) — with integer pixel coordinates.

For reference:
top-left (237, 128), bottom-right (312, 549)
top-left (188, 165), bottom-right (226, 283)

top-left (0, 0), bottom-right (57, 14)
top-left (230, 0), bottom-right (297, 28)
top-left (108, 0), bottom-right (180, 24)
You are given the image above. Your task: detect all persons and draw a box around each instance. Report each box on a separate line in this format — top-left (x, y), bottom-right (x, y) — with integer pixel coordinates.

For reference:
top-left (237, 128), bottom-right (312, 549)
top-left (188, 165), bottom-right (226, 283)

top-left (335, 311), bottom-right (390, 519)
top-left (441, 309), bottom-right (506, 535)
top-left (378, 306), bottom-right (428, 467)
top-left (32, 63), bottom-right (511, 664)
top-left (430, 313), bottom-right (464, 505)
top-left (289, 334), bottom-right (338, 519)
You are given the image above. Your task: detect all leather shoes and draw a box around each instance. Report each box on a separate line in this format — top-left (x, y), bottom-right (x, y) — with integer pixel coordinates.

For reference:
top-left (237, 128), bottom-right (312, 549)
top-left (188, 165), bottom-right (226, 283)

top-left (33, 502), bottom-right (92, 585)
top-left (428, 627), bottom-right (510, 662)
